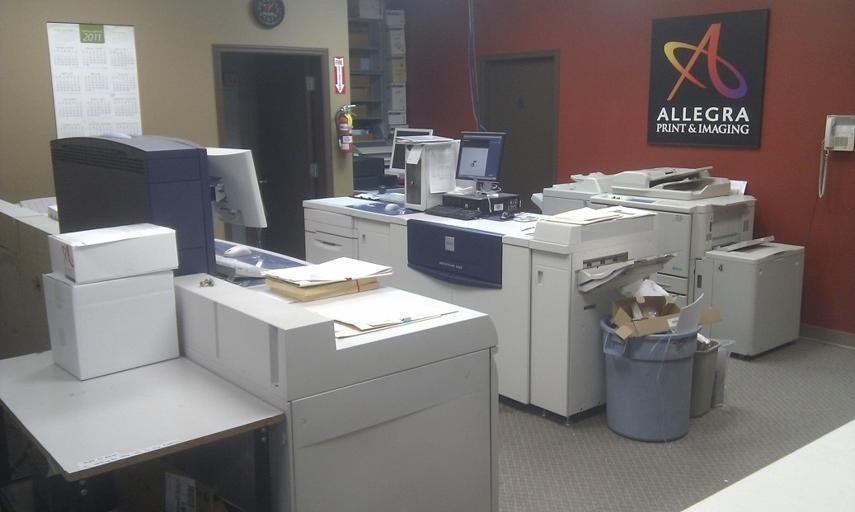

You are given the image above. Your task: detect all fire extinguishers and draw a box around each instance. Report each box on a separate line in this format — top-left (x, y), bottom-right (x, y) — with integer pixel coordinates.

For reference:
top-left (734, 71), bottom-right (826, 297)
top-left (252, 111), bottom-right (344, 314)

top-left (335, 104), bottom-right (356, 153)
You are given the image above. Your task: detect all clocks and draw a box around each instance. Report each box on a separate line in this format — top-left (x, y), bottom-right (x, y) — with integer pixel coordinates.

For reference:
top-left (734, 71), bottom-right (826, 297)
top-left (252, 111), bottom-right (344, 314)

top-left (253, 0), bottom-right (285, 28)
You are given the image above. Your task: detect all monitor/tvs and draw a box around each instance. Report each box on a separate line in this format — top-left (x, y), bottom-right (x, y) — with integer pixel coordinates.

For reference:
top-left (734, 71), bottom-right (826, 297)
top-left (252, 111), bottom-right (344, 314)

top-left (456, 131), bottom-right (508, 194)
top-left (205, 147), bottom-right (268, 228)
top-left (388, 127), bottom-right (434, 174)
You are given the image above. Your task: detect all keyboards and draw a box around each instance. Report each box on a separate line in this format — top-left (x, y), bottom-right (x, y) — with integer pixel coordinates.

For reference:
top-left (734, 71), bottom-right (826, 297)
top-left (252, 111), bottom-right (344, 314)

top-left (215, 254), bottom-right (266, 283)
top-left (423, 204), bottom-right (484, 220)
top-left (375, 193), bottom-right (405, 203)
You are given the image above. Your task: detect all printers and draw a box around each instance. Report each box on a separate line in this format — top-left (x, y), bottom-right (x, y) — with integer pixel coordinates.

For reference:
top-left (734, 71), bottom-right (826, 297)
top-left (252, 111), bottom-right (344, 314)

top-left (531, 166), bottom-right (806, 358)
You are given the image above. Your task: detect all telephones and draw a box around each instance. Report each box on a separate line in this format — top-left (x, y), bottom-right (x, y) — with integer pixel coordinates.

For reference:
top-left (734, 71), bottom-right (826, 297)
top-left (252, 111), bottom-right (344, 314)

top-left (823, 114), bottom-right (855, 152)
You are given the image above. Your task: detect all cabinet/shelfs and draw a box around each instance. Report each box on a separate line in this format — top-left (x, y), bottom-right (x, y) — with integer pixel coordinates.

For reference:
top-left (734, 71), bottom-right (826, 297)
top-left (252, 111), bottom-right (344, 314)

top-left (348, 18), bottom-right (386, 145)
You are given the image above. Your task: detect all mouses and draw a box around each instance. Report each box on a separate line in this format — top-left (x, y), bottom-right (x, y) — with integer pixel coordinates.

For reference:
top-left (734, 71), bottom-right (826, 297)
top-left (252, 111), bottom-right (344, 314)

top-left (384, 203), bottom-right (401, 212)
top-left (223, 244), bottom-right (252, 258)
top-left (500, 211), bottom-right (516, 220)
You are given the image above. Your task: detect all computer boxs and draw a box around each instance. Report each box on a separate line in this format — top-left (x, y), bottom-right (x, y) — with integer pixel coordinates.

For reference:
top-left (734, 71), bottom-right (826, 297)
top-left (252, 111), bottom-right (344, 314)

top-left (442, 192), bottom-right (522, 219)
top-left (404, 140), bottom-right (474, 213)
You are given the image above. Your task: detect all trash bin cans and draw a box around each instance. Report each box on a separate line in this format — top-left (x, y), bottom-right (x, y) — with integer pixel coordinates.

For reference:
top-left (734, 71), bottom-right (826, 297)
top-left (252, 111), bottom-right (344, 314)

top-left (712, 339), bottom-right (735, 408)
top-left (599, 315), bottom-right (702, 442)
top-left (689, 338), bottom-right (721, 417)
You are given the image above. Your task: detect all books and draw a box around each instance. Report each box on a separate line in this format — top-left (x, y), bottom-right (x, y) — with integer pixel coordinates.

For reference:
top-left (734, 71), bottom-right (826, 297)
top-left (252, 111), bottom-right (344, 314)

top-left (266, 262), bottom-right (380, 299)
